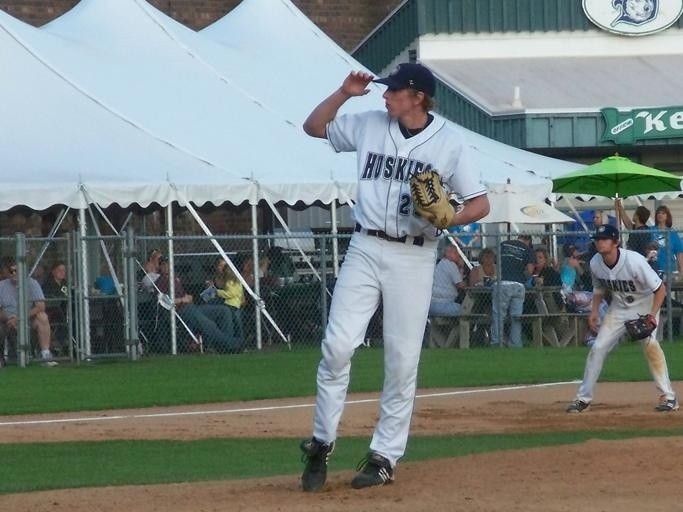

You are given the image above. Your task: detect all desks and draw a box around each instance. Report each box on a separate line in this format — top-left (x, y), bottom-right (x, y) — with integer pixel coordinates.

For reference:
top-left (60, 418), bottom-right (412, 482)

top-left (0, 265), bottom-right (683, 369)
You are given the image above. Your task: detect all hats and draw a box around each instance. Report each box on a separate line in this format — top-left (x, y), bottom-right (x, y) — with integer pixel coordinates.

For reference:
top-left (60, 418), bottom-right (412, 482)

top-left (589, 224), bottom-right (620, 240)
top-left (374, 62), bottom-right (435, 98)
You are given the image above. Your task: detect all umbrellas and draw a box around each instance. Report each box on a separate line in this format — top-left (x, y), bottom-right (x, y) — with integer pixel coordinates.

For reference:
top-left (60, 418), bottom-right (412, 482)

top-left (551, 151), bottom-right (683, 247)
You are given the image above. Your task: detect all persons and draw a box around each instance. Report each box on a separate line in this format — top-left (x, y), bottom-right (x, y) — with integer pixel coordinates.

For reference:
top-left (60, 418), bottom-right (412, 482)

top-left (298, 61), bottom-right (491, 493)
top-left (1, 246), bottom-right (335, 369)
top-left (423, 198), bottom-right (683, 348)
top-left (566, 223), bottom-right (679, 414)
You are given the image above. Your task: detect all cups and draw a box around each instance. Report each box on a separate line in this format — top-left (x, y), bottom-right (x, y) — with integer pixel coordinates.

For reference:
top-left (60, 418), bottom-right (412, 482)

top-left (94, 276), bottom-right (114, 296)
top-left (531, 274), bottom-right (544, 288)
top-left (278, 277), bottom-right (284, 287)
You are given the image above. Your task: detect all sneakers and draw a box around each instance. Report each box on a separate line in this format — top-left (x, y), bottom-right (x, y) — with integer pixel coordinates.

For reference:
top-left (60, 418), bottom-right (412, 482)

top-left (350, 450), bottom-right (394, 488)
top-left (566, 397), bottom-right (592, 413)
top-left (299, 436), bottom-right (336, 491)
top-left (653, 397), bottom-right (680, 412)
top-left (39, 349), bottom-right (58, 367)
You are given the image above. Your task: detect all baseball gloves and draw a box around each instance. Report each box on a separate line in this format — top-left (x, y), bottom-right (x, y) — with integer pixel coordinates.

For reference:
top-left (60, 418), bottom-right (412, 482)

top-left (624, 313), bottom-right (657, 340)
top-left (408, 170), bottom-right (454, 237)
